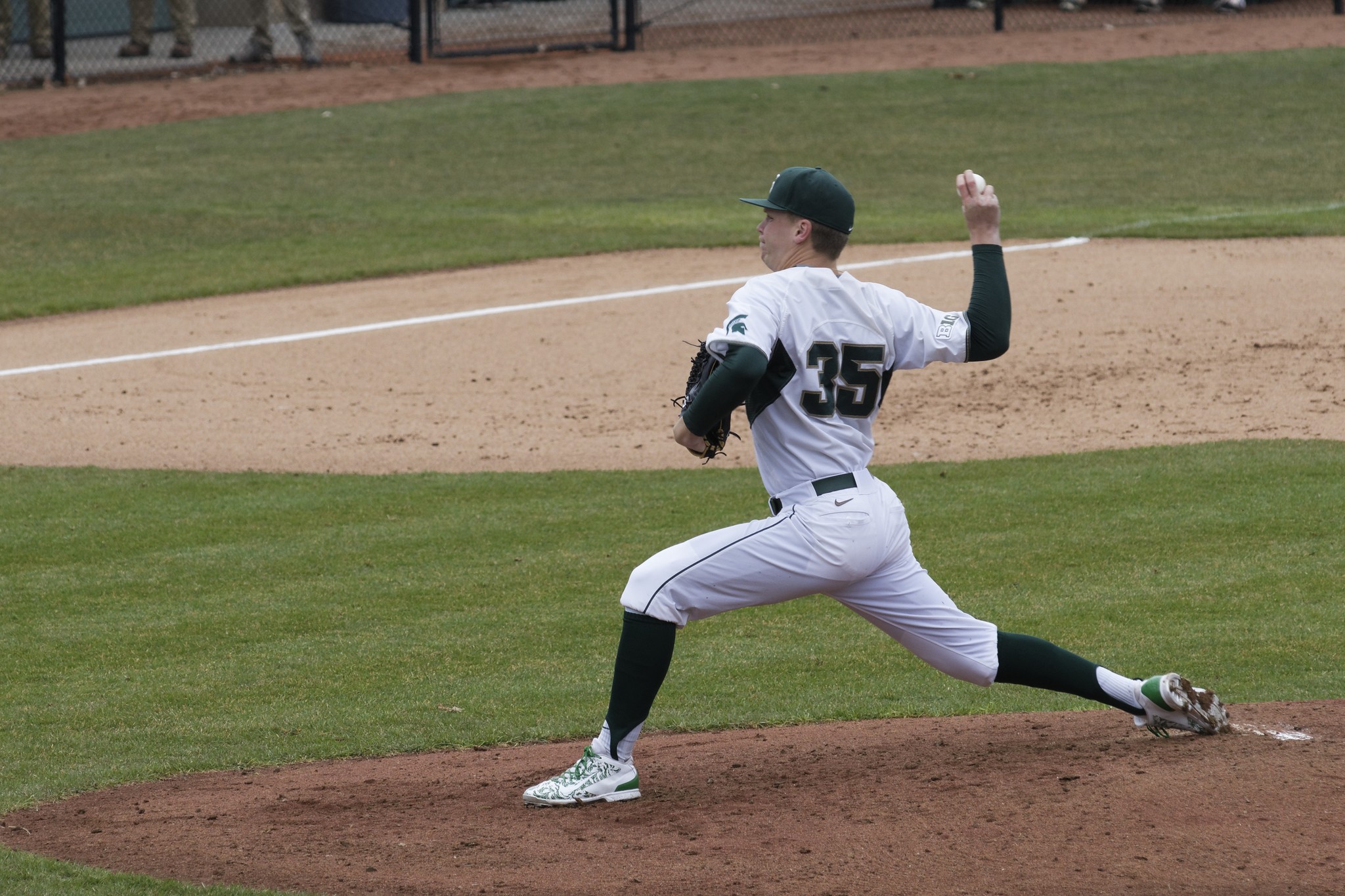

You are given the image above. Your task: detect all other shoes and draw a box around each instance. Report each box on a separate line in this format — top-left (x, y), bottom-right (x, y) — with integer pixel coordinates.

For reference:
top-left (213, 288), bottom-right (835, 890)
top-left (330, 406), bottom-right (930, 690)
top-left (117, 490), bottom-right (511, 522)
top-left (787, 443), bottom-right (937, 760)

top-left (301, 46), bottom-right (322, 68)
top-left (118, 38), bottom-right (150, 57)
top-left (169, 41), bottom-right (193, 57)
top-left (28, 41), bottom-right (55, 58)
top-left (229, 41), bottom-right (275, 63)
top-left (0, 49), bottom-right (11, 61)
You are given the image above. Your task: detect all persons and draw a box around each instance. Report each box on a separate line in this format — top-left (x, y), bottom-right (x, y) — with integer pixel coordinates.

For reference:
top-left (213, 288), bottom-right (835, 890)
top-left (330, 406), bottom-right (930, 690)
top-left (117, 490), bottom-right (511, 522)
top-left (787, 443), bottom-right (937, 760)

top-left (228, 0), bottom-right (321, 64)
top-left (521, 168), bottom-right (1231, 808)
top-left (118, 0), bottom-right (195, 58)
top-left (0, 0), bottom-right (53, 60)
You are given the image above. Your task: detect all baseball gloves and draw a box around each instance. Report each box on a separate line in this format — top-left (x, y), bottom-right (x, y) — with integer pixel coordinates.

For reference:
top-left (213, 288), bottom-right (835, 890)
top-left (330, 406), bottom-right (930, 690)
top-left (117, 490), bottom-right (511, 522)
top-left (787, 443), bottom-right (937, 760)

top-left (680, 340), bottom-right (732, 459)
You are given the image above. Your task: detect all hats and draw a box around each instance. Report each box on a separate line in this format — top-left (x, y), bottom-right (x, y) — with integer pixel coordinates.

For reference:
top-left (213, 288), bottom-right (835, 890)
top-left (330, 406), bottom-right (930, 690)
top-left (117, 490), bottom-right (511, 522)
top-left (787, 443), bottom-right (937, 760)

top-left (739, 167), bottom-right (855, 234)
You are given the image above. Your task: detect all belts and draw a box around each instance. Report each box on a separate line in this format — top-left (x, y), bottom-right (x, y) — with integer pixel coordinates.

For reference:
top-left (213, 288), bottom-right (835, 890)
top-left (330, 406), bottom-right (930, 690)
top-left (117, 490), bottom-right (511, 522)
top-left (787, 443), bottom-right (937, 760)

top-left (770, 469), bottom-right (874, 516)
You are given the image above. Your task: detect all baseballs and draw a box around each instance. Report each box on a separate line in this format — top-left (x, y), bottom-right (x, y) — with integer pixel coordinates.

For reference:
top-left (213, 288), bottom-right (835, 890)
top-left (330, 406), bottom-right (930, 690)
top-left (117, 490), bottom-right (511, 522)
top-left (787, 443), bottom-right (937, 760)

top-left (956, 173), bottom-right (985, 197)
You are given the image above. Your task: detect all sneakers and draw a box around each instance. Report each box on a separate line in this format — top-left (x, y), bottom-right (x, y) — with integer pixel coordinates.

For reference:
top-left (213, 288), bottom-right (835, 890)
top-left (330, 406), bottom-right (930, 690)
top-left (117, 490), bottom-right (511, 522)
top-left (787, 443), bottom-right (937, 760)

top-left (1133, 673), bottom-right (1229, 736)
top-left (522, 739), bottom-right (641, 806)
